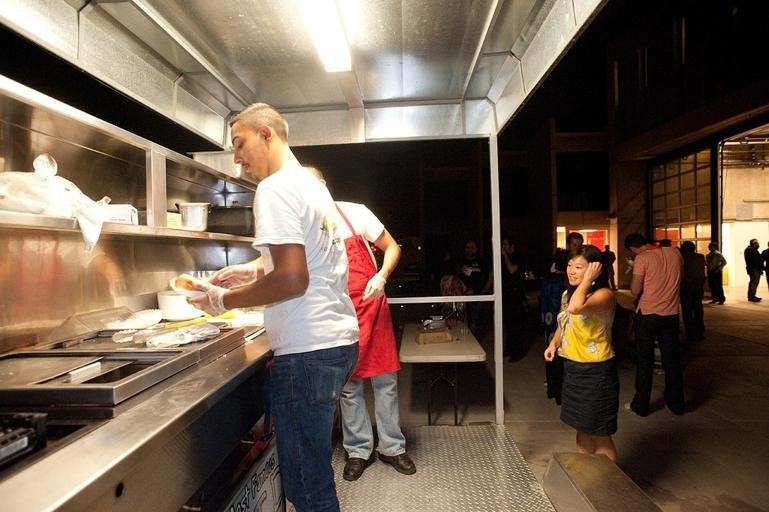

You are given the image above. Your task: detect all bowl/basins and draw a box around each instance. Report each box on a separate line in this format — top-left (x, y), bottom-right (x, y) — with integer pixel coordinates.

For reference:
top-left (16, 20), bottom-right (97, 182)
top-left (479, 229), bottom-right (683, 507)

top-left (179, 202), bottom-right (208, 232)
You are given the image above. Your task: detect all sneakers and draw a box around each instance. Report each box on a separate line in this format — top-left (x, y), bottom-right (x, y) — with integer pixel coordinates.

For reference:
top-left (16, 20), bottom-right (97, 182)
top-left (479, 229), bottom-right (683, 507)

top-left (710, 297), bottom-right (725, 305)
top-left (748, 295), bottom-right (762, 301)
top-left (624, 403), bottom-right (647, 417)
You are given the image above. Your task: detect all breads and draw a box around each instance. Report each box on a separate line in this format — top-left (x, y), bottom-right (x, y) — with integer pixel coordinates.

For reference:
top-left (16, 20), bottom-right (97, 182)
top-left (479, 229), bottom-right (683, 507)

top-left (174, 272), bottom-right (216, 295)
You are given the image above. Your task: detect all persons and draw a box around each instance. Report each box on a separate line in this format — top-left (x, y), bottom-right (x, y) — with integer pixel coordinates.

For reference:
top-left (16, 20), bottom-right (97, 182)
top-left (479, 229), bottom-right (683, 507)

top-left (543, 243), bottom-right (620, 464)
top-left (622, 233), bottom-right (688, 415)
top-left (302, 164), bottom-right (417, 482)
top-left (454, 232), bottom-right (769, 406)
top-left (186, 102), bottom-right (360, 512)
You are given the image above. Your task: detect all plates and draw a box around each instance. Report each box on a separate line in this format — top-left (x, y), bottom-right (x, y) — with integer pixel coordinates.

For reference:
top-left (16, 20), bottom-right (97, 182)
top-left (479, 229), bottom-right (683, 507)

top-left (123, 277), bottom-right (204, 328)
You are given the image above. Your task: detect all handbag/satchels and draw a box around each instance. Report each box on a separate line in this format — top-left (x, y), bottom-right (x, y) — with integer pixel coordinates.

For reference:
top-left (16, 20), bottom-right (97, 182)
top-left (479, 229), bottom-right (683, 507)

top-left (546, 357), bottom-right (565, 405)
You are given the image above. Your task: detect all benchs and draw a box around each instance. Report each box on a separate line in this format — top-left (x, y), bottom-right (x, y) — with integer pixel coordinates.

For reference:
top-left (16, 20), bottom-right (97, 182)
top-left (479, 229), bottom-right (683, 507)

top-left (540, 451), bottom-right (666, 512)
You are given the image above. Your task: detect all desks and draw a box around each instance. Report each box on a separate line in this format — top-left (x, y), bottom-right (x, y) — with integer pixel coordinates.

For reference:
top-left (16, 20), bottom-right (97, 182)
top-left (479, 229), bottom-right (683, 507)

top-left (398, 319), bottom-right (486, 425)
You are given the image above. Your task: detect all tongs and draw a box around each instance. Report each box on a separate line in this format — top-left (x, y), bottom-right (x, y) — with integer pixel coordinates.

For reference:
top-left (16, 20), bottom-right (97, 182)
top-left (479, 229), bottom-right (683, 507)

top-left (111, 325), bottom-right (178, 345)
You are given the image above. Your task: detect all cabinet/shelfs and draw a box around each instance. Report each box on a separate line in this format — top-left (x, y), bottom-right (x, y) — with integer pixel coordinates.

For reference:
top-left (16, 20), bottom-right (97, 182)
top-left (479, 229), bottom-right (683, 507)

top-left (0, 73), bottom-right (257, 242)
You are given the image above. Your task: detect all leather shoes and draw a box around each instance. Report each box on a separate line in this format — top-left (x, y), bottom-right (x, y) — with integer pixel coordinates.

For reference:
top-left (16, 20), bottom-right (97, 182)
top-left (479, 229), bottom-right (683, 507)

top-left (343, 450), bottom-right (375, 480)
top-left (378, 451), bottom-right (416, 474)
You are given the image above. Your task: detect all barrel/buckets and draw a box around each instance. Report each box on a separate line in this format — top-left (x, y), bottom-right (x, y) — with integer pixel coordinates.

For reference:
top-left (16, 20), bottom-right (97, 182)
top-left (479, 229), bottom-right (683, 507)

top-left (175, 202), bottom-right (212, 232)
